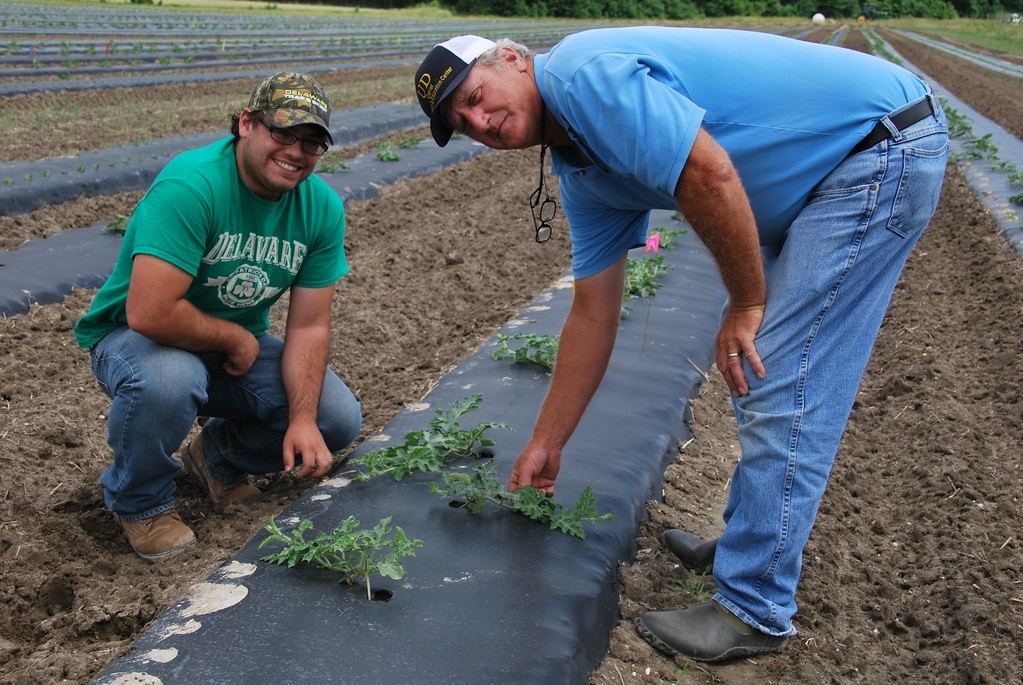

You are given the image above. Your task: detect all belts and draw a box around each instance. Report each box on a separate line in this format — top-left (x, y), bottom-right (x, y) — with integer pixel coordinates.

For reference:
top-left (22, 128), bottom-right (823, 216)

top-left (846, 96), bottom-right (939, 157)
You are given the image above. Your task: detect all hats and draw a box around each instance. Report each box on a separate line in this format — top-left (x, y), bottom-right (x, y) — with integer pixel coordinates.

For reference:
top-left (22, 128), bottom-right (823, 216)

top-left (248, 72), bottom-right (335, 145)
top-left (415, 35), bottom-right (494, 148)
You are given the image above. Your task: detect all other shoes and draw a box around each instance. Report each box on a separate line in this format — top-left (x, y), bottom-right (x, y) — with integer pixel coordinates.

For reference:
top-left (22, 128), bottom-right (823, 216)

top-left (635, 600), bottom-right (789, 662)
top-left (661, 529), bottom-right (721, 576)
top-left (181, 430), bottom-right (262, 515)
top-left (112, 507), bottom-right (197, 565)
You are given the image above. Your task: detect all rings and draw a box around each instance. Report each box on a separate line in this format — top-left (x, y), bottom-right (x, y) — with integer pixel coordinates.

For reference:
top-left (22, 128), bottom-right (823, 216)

top-left (727, 353), bottom-right (739, 357)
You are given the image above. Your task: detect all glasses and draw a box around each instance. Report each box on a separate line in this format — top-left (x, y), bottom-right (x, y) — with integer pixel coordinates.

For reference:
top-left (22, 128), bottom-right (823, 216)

top-left (529, 142), bottom-right (557, 244)
top-left (257, 117), bottom-right (328, 155)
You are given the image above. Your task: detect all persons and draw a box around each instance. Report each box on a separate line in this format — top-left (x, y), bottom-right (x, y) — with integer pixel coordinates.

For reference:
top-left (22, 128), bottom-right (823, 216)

top-left (73, 70), bottom-right (363, 561)
top-left (415, 25), bottom-right (950, 660)
